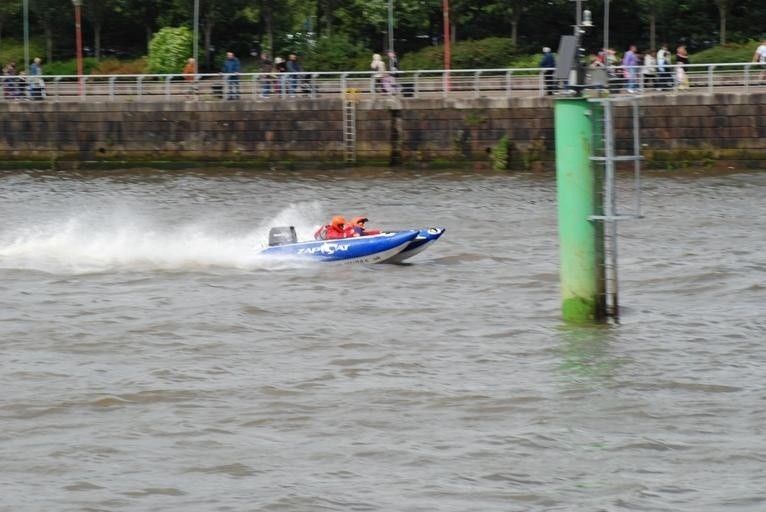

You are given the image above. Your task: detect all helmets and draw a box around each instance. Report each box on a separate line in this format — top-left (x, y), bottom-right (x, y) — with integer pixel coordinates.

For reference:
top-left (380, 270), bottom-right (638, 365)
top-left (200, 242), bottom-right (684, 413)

top-left (351, 216), bottom-right (368, 230)
top-left (332, 215), bottom-right (347, 233)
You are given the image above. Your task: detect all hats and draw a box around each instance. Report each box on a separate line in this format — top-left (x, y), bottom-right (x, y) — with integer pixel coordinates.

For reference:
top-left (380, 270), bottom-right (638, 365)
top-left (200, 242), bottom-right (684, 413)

top-left (543, 47), bottom-right (552, 53)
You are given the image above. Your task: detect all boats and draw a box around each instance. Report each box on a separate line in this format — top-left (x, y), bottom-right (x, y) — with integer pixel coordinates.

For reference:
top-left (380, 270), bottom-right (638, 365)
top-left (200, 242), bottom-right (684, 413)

top-left (258, 226), bottom-right (446, 265)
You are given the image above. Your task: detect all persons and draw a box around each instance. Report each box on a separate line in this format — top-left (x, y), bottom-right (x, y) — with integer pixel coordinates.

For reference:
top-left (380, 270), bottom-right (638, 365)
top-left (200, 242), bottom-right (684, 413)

top-left (751, 40), bottom-right (766, 86)
top-left (0, 55), bottom-right (45, 100)
top-left (184, 47), bottom-right (403, 98)
top-left (537, 41), bottom-right (695, 96)
top-left (346, 216), bottom-right (383, 238)
top-left (311, 214), bottom-right (349, 239)
